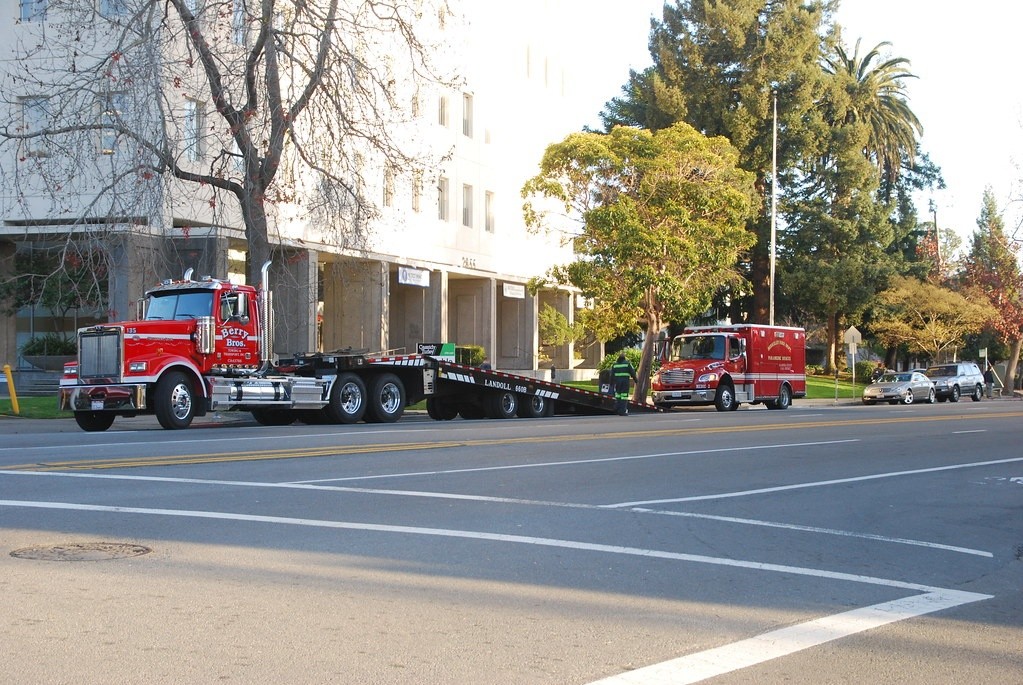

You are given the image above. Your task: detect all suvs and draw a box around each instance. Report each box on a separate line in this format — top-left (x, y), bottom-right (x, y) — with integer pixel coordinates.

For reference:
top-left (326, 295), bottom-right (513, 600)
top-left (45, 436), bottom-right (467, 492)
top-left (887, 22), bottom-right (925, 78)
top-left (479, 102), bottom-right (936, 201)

top-left (923, 360), bottom-right (987, 404)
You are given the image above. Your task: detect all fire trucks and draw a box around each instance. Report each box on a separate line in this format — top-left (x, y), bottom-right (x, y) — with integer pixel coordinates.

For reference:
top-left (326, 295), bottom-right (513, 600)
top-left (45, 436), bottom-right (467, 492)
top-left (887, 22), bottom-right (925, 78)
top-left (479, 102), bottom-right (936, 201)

top-left (651, 322), bottom-right (807, 413)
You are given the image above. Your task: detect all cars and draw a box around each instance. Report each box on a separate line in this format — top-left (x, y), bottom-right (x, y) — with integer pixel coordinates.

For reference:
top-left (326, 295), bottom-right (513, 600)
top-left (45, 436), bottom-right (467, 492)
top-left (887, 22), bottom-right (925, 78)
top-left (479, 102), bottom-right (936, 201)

top-left (862, 371), bottom-right (937, 406)
top-left (909, 368), bottom-right (927, 376)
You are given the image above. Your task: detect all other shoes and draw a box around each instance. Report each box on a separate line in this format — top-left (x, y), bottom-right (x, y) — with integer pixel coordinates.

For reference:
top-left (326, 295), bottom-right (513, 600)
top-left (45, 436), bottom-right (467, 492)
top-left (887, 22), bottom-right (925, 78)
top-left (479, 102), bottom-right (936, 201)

top-left (619, 412), bottom-right (628, 416)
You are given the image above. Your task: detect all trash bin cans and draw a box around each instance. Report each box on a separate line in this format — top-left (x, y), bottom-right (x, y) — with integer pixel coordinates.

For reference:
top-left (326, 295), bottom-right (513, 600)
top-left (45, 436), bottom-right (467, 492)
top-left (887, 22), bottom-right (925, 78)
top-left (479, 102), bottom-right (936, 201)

top-left (599, 370), bottom-right (615, 397)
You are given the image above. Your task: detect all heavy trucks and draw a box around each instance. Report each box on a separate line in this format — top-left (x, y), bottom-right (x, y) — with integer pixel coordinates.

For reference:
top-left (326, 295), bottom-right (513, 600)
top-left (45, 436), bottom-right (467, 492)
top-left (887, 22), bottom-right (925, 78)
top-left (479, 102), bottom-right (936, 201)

top-left (58, 260), bottom-right (673, 433)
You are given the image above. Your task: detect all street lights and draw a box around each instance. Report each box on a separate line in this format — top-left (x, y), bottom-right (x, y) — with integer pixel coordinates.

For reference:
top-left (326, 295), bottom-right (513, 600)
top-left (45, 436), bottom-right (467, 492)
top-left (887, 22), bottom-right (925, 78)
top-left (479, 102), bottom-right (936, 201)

top-left (770, 80), bottom-right (784, 326)
top-left (929, 198), bottom-right (942, 279)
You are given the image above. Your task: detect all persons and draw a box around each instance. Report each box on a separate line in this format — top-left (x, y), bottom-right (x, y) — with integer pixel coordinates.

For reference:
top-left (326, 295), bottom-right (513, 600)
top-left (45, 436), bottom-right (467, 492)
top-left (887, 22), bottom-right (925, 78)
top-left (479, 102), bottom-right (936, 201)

top-left (610, 354), bottom-right (639, 416)
top-left (871, 363), bottom-right (885, 384)
top-left (983, 366), bottom-right (993, 398)
top-left (551, 363), bottom-right (556, 383)
top-left (481, 356), bottom-right (491, 370)
